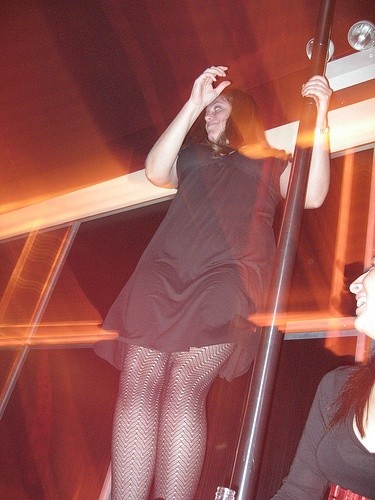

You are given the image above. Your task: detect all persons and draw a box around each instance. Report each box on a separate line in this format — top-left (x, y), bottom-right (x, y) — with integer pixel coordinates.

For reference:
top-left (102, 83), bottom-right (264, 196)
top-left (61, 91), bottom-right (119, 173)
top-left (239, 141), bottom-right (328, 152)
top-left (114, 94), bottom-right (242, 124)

top-left (91, 63), bottom-right (335, 499)
top-left (267, 249), bottom-right (375, 499)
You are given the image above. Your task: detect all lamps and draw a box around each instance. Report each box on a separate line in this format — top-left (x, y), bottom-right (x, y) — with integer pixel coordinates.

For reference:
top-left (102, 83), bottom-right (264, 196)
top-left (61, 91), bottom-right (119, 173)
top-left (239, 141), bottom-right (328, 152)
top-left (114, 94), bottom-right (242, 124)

top-left (304, 34), bottom-right (335, 64)
top-left (345, 19), bottom-right (374, 53)
